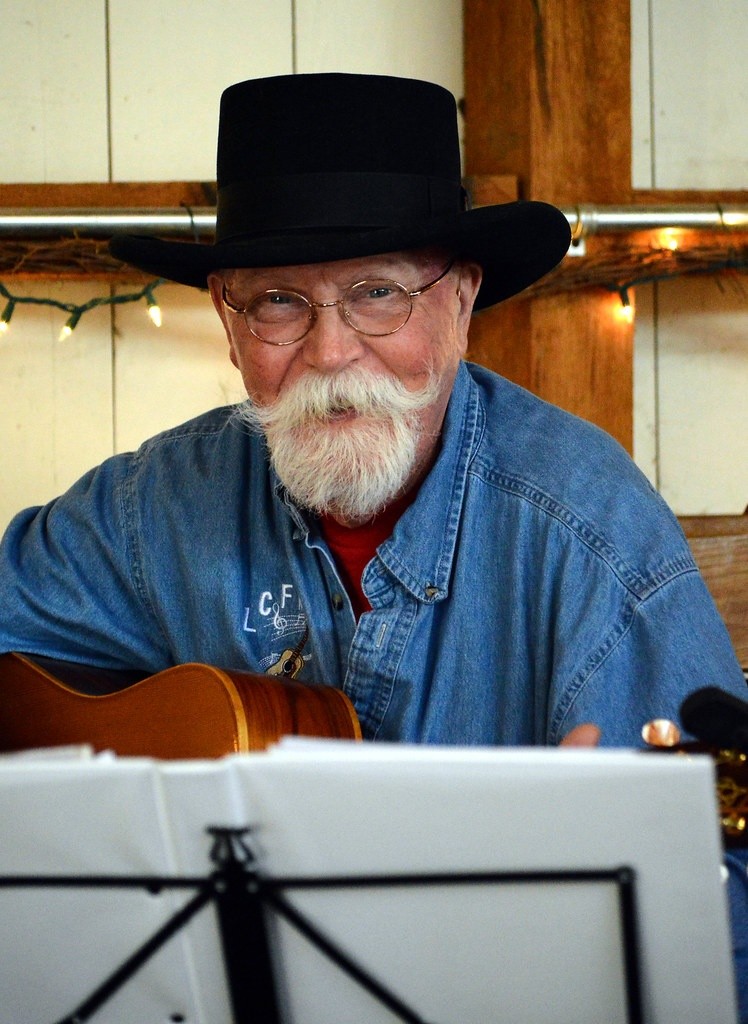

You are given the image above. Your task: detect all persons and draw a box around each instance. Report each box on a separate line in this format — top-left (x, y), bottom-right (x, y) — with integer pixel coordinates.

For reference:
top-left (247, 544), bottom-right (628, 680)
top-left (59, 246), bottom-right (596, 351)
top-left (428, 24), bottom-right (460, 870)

top-left (0, 73), bottom-right (747, 748)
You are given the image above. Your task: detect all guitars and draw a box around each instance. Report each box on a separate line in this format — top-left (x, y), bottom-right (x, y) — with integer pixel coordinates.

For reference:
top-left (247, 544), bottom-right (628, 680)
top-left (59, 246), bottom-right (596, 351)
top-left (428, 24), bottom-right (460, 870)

top-left (1, 646), bottom-right (747, 844)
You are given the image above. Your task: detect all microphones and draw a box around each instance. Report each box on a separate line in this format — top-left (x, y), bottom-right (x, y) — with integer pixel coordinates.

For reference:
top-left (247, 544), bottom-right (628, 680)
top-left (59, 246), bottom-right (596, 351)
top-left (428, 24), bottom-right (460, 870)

top-left (680, 687), bottom-right (747, 760)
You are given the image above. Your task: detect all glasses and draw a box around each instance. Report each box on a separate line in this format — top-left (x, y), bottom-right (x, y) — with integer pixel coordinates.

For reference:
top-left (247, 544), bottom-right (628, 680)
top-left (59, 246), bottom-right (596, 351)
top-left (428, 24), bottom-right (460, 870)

top-left (222, 258), bottom-right (462, 347)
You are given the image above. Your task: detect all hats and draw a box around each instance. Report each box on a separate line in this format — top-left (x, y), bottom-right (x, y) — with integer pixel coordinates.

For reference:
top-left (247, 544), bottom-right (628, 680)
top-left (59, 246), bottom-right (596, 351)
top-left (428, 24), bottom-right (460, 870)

top-left (107, 71), bottom-right (573, 315)
